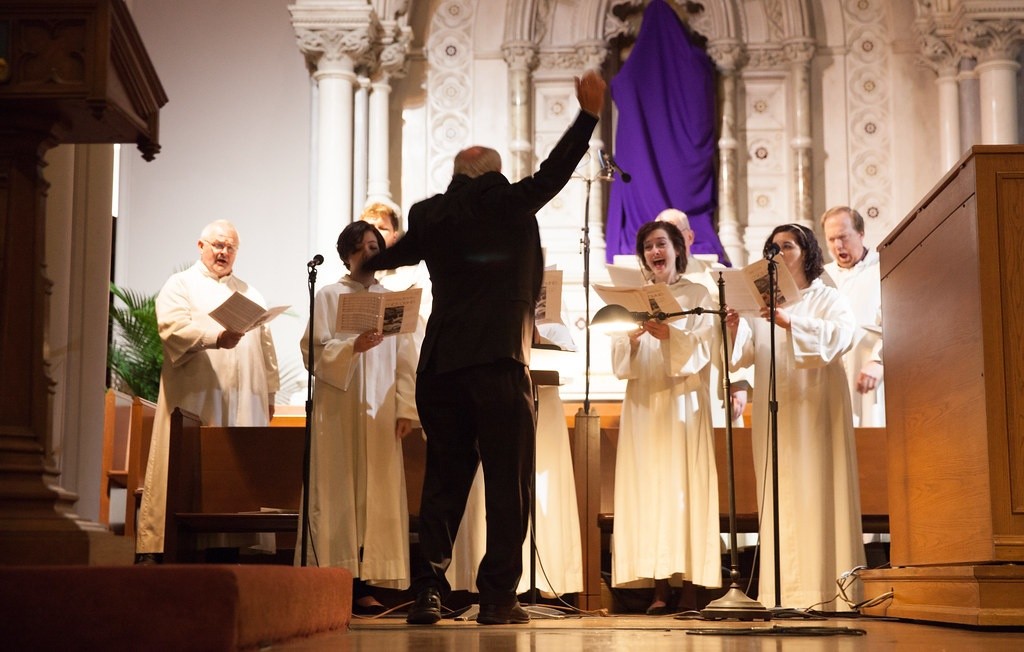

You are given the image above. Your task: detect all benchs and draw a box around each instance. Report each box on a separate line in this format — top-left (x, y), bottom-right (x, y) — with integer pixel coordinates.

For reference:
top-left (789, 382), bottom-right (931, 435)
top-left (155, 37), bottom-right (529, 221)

top-left (587, 422), bottom-right (901, 609)
top-left (163, 405), bottom-right (417, 570)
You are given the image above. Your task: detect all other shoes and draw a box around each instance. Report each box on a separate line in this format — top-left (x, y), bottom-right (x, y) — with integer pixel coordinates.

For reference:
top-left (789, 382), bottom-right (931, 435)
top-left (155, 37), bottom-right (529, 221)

top-left (645, 601), bottom-right (673, 615)
top-left (353, 599), bottom-right (390, 616)
top-left (676, 604), bottom-right (697, 613)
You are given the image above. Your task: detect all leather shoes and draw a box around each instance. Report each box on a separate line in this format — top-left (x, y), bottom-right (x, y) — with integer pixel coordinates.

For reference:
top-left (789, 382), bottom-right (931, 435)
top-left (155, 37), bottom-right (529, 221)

top-left (475, 600), bottom-right (532, 625)
top-left (405, 587), bottom-right (442, 625)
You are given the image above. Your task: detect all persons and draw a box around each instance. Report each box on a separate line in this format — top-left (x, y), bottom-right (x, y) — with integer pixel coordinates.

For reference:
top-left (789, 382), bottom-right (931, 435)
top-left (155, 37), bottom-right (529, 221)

top-left (293, 195), bottom-right (583, 625)
top-left (610, 206), bottom-right (886, 623)
top-left (348, 65), bottom-right (607, 630)
top-left (132, 215), bottom-right (284, 569)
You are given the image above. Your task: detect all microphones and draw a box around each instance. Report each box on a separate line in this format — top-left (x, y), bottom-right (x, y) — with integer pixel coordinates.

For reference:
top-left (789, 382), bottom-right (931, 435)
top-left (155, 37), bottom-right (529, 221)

top-left (308, 254), bottom-right (324, 267)
top-left (600, 150), bottom-right (632, 183)
top-left (766, 243), bottom-right (780, 260)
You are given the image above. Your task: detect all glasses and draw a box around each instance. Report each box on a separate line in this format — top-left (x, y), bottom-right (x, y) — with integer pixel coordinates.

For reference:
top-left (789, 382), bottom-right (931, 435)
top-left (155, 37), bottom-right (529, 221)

top-left (201, 238), bottom-right (238, 254)
